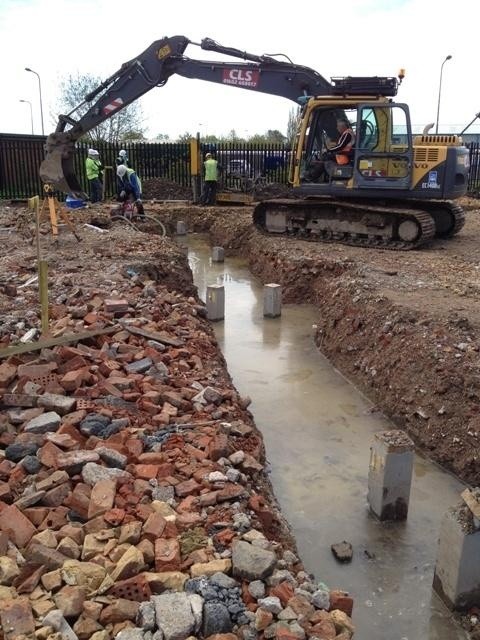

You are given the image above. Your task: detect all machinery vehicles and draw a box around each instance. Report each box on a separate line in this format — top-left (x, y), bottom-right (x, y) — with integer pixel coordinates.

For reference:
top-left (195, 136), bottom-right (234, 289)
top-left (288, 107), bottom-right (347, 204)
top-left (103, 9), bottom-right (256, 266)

top-left (39, 35), bottom-right (471, 251)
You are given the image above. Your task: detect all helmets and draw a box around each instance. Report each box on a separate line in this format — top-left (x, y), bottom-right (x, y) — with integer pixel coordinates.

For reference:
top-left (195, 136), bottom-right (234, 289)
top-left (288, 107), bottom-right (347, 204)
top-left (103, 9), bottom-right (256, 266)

top-left (119, 150), bottom-right (126, 156)
top-left (88, 149), bottom-right (100, 155)
top-left (117, 165), bottom-right (126, 176)
top-left (206, 153), bottom-right (212, 157)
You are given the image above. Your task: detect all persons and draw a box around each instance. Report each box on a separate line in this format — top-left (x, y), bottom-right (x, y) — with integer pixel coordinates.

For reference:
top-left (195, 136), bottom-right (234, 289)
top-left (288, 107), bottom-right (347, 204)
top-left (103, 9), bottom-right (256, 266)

top-left (303, 118), bottom-right (356, 182)
top-left (117, 165), bottom-right (147, 222)
top-left (95, 150), bottom-right (104, 184)
top-left (86, 148), bottom-right (104, 203)
top-left (116, 149), bottom-right (132, 194)
top-left (199, 153), bottom-right (227, 206)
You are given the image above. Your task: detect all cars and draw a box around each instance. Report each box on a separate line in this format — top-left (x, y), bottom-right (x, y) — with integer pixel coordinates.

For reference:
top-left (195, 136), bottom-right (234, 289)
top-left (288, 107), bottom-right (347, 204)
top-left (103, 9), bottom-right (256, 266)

top-left (226, 159), bottom-right (251, 178)
top-left (263, 156), bottom-right (284, 175)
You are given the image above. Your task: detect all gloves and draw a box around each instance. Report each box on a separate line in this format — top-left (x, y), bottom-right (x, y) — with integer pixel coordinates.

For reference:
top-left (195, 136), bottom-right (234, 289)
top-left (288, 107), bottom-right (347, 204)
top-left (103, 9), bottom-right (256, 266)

top-left (120, 191), bottom-right (126, 199)
top-left (137, 199), bottom-right (142, 205)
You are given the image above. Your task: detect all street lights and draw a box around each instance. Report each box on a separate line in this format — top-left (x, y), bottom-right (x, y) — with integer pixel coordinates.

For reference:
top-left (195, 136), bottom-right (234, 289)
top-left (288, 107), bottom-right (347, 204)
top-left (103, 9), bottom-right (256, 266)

top-left (25, 68), bottom-right (44, 136)
top-left (19, 100), bottom-right (33, 136)
top-left (436, 54), bottom-right (452, 134)
